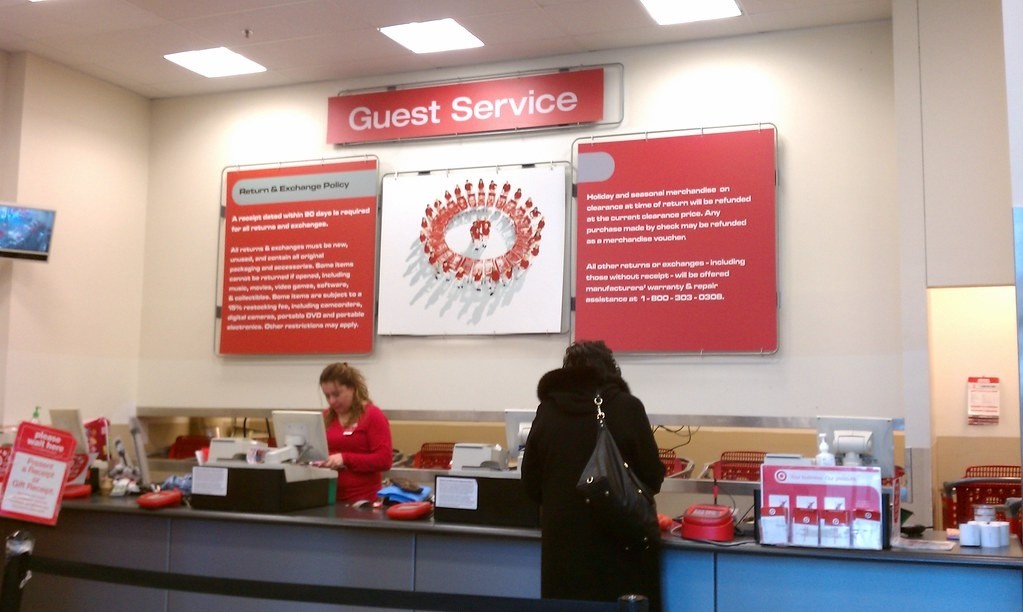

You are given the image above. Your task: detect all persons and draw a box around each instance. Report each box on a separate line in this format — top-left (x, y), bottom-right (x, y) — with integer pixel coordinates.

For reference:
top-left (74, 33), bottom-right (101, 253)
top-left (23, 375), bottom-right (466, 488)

top-left (519, 340), bottom-right (660, 612)
top-left (319, 362), bottom-right (393, 503)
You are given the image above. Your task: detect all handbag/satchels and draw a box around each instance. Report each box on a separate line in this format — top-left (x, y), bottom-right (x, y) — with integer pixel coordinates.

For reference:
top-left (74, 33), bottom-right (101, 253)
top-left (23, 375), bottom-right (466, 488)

top-left (576, 388), bottom-right (661, 565)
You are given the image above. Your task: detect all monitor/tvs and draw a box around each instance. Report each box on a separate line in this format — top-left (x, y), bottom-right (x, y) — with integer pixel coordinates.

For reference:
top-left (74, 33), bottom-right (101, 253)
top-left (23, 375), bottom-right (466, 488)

top-left (49, 409), bottom-right (89, 454)
top-left (272, 411), bottom-right (330, 466)
top-left (0, 203), bottom-right (55, 262)
top-left (505, 410), bottom-right (537, 456)
top-left (816, 415), bottom-right (895, 478)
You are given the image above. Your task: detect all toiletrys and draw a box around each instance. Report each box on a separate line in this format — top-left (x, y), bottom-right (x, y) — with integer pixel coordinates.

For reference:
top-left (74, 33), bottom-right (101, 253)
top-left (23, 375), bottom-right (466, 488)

top-left (814, 432), bottom-right (837, 468)
